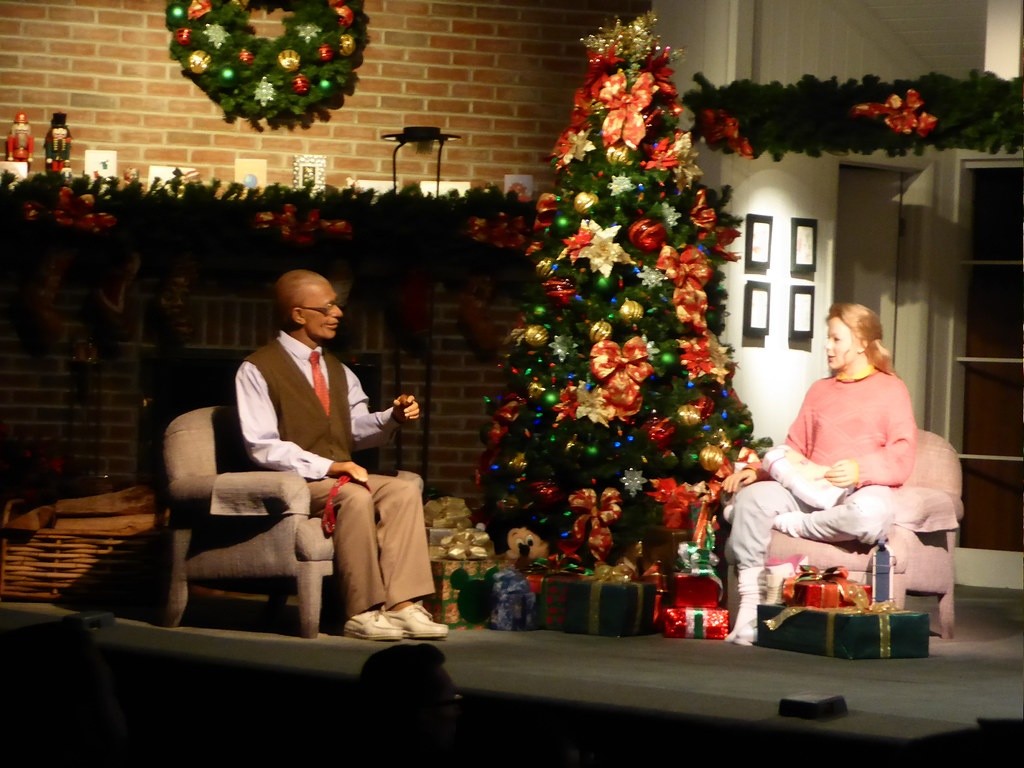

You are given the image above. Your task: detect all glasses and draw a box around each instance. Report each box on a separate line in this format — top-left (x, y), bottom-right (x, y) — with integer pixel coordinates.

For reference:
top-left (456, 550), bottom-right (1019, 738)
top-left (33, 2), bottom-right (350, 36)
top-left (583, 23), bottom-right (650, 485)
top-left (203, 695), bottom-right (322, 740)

top-left (296, 300), bottom-right (344, 317)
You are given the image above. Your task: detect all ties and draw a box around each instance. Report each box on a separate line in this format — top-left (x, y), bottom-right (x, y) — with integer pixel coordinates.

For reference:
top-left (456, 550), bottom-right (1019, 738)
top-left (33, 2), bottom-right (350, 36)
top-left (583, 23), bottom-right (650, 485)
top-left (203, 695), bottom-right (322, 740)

top-left (309, 351), bottom-right (330, 415)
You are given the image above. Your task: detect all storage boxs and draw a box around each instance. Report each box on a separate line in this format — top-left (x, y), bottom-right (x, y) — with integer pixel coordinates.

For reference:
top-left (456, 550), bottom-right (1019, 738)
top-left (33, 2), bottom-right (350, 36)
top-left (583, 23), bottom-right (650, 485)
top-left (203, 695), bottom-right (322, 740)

top-left (755, 603), bottom-right (931, 661)
top-left (424, 525), bottom-right (729, 641)
top-left (783, 576), bottom-right (871, 609)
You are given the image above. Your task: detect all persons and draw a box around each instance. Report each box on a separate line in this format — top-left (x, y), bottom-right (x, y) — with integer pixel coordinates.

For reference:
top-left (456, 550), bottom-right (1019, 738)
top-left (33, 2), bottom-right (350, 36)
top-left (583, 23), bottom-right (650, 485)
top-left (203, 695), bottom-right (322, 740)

top-left (721, 302), bottom-right (916, 645)
top-left (236, 271), bottom-right (450, 642)
top-left (358, 645), bottom-right (460, 768)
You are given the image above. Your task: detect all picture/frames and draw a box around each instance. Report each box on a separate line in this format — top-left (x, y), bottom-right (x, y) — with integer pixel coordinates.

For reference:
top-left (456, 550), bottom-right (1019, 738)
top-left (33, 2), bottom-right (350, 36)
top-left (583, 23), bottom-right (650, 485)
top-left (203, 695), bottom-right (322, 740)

top-left (787, 284), bottom-right (815, 339)
top-left (742, 280), bottom-right (771, 338)
top-left (745, 213), bottom-right (773, 269)
top-left (790, 216), bottom-right (819, 274)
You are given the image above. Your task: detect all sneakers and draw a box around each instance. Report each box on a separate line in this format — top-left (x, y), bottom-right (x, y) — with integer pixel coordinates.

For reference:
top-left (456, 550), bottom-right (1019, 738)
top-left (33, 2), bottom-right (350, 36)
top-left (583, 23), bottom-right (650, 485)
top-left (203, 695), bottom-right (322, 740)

top-left (343, 606), bottom-right (403, 641)
top-left (385, 603), bottom-right (449, 639)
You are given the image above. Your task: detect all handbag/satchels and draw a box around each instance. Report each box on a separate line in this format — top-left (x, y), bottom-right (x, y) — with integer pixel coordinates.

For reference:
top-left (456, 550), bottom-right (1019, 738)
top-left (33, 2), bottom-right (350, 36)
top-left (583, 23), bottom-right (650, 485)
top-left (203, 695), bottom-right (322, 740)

top-left (758, 443), bottom-right (855, 510)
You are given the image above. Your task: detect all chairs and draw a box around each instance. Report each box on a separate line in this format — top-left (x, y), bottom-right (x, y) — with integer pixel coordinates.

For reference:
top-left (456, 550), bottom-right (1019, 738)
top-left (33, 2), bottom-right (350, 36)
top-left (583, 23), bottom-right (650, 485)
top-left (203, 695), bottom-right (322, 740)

top-left (159, 403), bottom-right (423, 640)
top-left (723, 430), bottom-right (966, 638)
